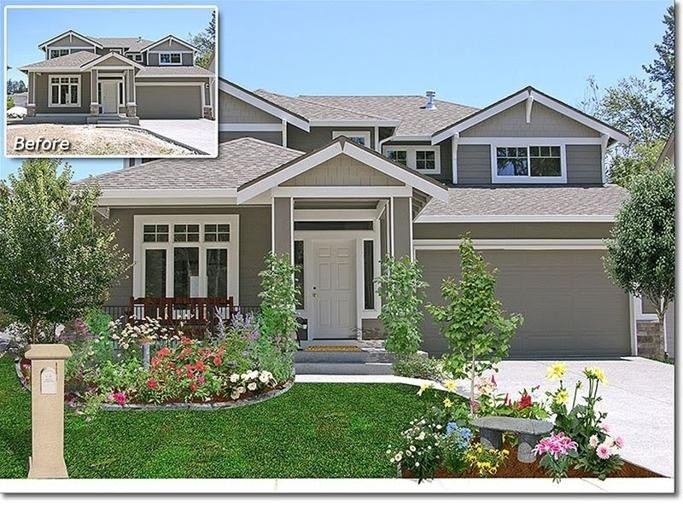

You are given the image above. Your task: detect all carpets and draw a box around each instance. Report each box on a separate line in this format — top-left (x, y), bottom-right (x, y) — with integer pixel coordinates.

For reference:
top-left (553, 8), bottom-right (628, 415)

top-left (304, 343), bottom-right (363, 354)
